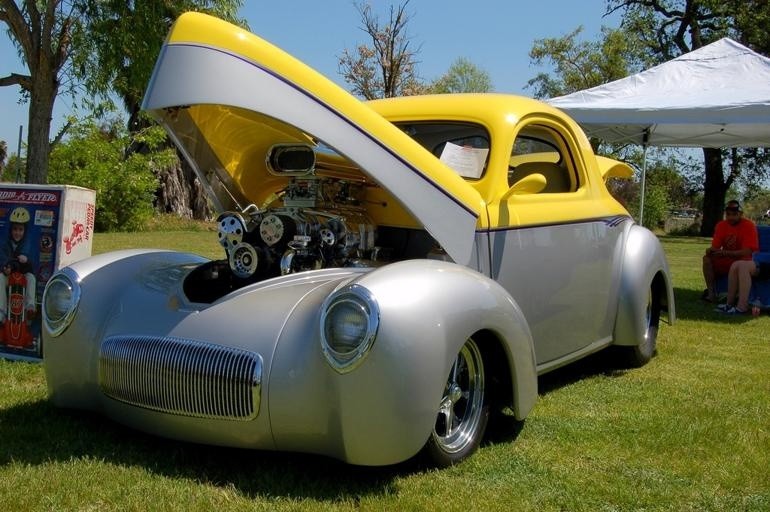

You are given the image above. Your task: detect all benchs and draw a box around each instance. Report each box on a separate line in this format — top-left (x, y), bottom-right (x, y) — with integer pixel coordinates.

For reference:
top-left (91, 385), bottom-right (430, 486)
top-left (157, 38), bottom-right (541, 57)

top-left (713, 225), bottom-right (770, 309)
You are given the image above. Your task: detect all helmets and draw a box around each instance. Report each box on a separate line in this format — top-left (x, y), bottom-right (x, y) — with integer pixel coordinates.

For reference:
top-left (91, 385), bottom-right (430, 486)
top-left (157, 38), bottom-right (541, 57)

top-left (9, 207), bottom-right (30, 223)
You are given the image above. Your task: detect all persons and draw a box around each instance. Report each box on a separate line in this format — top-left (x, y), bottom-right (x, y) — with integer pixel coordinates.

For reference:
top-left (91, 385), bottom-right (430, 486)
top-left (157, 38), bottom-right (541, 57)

top-left (700, 200), bottom-right (761, 305)
top-left (0, 206), bottom-right (38, 334)
top-left (713, 209), bottom-right (770, 316)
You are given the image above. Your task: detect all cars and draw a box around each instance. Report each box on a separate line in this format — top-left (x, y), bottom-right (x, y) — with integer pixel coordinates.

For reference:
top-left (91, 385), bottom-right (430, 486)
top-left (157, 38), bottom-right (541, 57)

top-left (41, 11), bottom-right (677, 469)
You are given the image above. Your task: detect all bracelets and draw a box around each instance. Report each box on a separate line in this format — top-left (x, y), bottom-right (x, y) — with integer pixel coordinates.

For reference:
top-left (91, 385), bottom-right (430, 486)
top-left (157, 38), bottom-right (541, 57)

top-left (721, 250), bottom-right (727, 257)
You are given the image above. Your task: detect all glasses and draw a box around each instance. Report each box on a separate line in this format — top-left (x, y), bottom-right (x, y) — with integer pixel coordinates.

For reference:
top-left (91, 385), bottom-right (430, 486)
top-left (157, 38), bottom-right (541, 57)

top-left (724, 206), bottom-right (739, 213)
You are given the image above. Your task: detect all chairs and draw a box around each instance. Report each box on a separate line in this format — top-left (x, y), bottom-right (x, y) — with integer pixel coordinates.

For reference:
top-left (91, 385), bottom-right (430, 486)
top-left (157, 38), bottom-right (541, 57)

top-left (508, 161), bottom-right (567, 193)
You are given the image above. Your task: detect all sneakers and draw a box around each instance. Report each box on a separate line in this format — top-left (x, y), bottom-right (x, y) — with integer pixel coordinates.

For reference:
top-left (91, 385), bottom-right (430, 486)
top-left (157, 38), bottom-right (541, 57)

top-left (713, 304), bottom-right (733, 313)
top-left (729, 306), bottom-right (750, 314)
